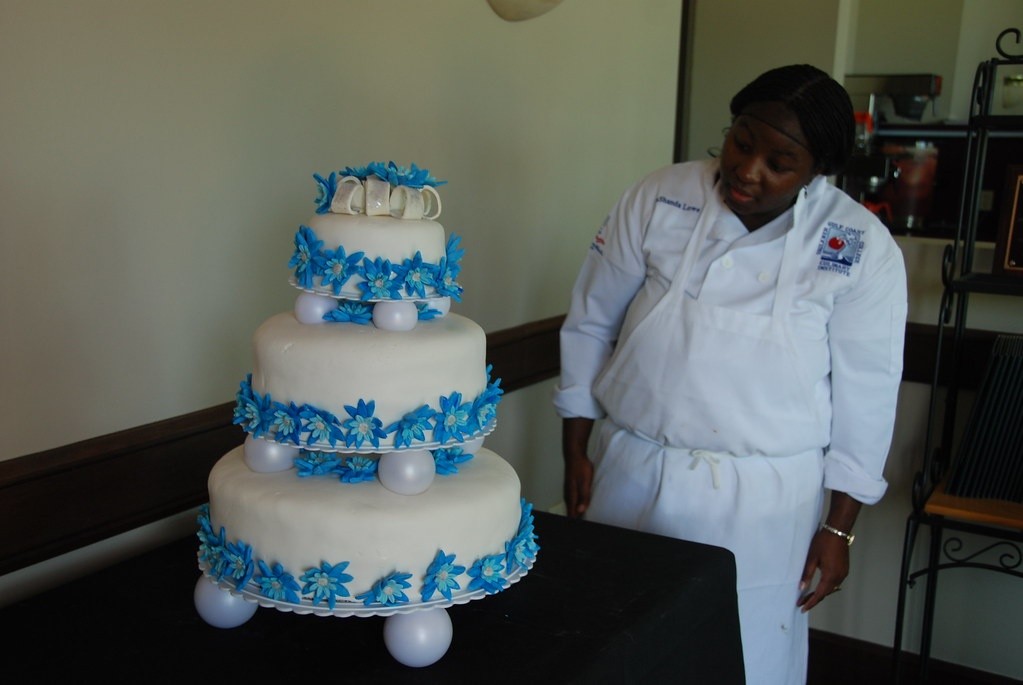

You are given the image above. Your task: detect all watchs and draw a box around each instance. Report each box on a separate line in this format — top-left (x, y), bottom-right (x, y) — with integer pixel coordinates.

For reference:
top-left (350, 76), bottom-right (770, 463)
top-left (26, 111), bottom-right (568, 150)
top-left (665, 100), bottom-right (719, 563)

top-left (820, 521), bottom-right (856, 547)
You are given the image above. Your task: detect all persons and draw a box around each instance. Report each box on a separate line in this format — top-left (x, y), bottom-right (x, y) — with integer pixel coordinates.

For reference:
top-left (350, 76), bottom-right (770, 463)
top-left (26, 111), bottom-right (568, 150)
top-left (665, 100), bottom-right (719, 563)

top-left (552, 66), bottom-right (905, 685)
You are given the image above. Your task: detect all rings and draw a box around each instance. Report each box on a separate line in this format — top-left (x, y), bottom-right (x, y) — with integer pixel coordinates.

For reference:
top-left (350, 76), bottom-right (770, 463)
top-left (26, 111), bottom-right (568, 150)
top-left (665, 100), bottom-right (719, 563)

top-left (833, 585), bottom-right (841, 592)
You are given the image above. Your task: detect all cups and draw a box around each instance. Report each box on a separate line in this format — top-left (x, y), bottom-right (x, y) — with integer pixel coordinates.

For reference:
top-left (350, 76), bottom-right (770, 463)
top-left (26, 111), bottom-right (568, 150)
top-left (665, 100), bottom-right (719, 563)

top-left (886, 139), bottom-right (939, 235)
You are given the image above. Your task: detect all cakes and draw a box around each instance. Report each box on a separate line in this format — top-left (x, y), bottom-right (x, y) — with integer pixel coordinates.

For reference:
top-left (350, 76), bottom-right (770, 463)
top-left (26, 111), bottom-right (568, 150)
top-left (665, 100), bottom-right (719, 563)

top-left (196, 159), bottom-right (540, 614)
